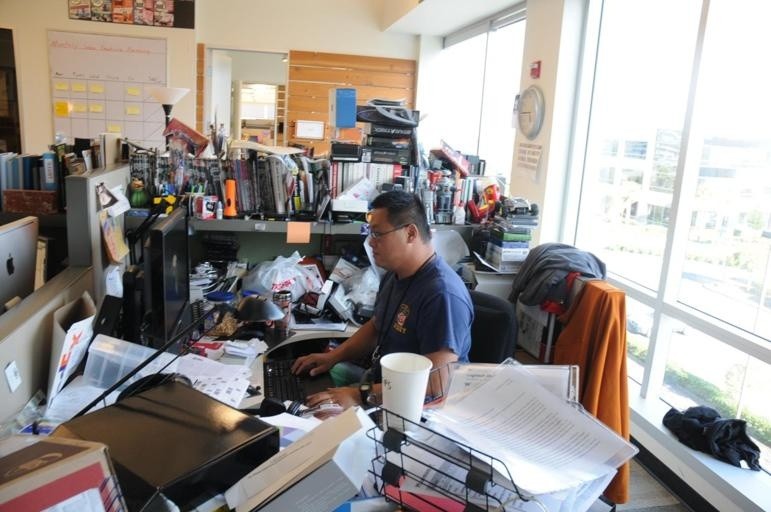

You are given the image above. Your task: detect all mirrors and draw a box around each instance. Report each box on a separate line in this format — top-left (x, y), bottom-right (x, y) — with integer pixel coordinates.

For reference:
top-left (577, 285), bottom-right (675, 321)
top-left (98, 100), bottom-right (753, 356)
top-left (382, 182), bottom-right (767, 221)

top-left (201, 47), bottom-right (288, 149)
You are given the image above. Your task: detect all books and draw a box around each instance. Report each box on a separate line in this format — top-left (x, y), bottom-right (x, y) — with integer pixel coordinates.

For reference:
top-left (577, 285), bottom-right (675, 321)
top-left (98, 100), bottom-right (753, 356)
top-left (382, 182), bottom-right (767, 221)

top-left (0, 152), bottom-right (61, 191)
top-left (131, 154), bottom-right (497, 225)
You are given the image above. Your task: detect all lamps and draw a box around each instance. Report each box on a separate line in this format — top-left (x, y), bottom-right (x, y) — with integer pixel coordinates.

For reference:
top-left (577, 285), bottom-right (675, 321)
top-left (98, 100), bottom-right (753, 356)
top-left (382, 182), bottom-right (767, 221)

top-left (149, 87), bottom-right (191, 151)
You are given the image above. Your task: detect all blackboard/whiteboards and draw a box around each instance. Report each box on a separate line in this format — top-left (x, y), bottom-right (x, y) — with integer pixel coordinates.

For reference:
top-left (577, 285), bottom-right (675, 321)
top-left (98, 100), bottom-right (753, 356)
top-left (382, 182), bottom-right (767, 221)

top-left (47, 29), bottom-right (171, 152)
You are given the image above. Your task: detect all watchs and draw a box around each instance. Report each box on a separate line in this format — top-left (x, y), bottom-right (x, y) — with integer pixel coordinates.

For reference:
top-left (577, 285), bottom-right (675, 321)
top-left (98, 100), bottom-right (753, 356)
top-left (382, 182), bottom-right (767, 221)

top-left (359, 382), bottom-right (373, 410)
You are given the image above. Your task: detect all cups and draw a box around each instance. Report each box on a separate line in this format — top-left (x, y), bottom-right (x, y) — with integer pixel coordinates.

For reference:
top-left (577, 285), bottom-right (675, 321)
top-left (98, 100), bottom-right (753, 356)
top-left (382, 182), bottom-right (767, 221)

top-left (379, 352), bottom-right (433, 433)
top-left (272, 291), bottom-right (292, 332)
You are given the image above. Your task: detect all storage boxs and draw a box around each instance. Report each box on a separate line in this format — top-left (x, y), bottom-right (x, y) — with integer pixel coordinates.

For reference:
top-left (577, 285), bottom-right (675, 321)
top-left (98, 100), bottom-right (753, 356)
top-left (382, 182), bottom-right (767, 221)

top-left (0, 432), bottom-right (129, 511)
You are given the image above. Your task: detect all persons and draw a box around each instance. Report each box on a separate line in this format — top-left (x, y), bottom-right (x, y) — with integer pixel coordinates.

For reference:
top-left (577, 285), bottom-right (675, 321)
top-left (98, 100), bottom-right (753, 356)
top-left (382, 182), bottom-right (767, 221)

top-left (291, 191), bottom-right (475, 410)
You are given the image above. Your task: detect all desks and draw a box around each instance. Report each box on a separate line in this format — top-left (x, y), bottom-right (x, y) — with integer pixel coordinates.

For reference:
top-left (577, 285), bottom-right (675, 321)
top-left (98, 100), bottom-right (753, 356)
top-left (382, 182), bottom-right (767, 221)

top-left (217, 324), bottom-right (360, 410)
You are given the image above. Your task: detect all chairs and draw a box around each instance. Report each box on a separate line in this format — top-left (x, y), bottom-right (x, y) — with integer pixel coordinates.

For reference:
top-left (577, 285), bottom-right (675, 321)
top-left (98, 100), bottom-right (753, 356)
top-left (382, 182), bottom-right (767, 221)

top-left (468, 290), bottom-right (517, 363)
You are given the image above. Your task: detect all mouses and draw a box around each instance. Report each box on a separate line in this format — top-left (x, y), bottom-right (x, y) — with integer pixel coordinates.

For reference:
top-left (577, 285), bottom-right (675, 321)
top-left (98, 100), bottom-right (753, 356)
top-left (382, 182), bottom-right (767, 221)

top-left (240, 329), bottom-right (264, 340)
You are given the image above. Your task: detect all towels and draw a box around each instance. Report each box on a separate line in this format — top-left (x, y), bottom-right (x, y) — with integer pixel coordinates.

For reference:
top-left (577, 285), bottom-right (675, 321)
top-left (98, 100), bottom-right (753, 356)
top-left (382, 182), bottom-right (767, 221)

top-left (553, 280), bottom-right (629, 504)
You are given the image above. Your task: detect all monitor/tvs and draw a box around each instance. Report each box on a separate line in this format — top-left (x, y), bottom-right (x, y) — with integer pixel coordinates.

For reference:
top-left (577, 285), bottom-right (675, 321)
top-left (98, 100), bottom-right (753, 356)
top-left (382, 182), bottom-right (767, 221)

top-left (0, 216), bottom-right (40, 316)
top-left (122, 206), bottom-right (192, 355)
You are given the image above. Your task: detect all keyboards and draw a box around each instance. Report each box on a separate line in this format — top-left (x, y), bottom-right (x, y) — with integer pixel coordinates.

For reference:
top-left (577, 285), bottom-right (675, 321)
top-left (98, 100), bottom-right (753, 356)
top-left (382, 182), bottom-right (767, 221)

top-left (264, 358), bottom-right (306, 403)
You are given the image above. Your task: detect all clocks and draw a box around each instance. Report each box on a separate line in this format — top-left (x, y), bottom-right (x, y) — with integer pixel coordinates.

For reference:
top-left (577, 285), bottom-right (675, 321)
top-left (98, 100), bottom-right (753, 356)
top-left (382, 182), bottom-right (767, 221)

top-left (518, 85), bottom-right (544, 139)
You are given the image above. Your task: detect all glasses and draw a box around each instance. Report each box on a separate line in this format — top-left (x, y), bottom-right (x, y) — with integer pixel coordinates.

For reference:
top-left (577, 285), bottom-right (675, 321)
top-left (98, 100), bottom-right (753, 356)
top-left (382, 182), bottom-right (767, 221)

top-left (370, 223), bottom-right (409, 239)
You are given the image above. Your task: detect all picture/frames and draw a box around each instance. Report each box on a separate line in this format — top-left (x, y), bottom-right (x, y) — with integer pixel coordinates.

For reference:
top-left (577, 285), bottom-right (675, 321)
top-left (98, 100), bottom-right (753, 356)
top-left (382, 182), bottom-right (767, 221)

top-left (295, 120), bottom-right (325, 140)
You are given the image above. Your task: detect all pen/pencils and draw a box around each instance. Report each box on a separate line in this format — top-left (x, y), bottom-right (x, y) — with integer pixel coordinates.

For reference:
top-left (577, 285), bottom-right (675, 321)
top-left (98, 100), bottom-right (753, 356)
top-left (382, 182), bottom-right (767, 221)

top-left (267, 218), bottom-right (285, 221)
top-left (285, 217), bottom-right (316, 221)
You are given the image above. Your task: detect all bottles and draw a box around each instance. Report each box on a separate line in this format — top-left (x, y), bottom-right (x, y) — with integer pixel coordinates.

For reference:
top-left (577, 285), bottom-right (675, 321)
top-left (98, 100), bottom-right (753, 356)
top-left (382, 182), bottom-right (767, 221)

top-left (421, 179), bottom-right (436, 224)
top-left (436, 184), bottom-right (453, 224)
top-left (216, 201), bottom-right (223, 220)
top-left (455, 200), bottom-right (465, 226)
top-left (205, 291), bottom-right (237, 336)
top-left (240, 289), bottom-right (261, 302)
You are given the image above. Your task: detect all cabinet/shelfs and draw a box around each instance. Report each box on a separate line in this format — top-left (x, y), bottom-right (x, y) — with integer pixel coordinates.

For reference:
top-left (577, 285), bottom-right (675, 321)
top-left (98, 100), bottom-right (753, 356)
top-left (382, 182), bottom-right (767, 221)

top-left (0, 28), bottom-right (21, 154)
